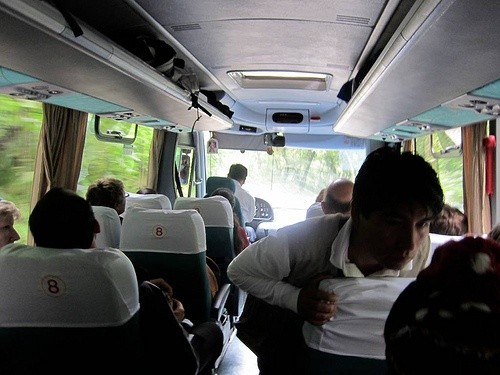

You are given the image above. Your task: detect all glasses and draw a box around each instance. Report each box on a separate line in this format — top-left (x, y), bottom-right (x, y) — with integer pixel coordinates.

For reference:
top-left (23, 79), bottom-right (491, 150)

top-left (120, 192), bottom-right (129, 201)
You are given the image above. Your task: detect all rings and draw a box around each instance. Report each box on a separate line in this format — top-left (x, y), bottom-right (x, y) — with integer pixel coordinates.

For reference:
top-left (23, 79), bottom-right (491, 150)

top-left (328, 318), bottom-right (333, 322)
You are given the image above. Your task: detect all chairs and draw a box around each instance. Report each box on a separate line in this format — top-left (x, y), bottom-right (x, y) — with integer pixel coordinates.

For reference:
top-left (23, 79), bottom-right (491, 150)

top-left (0, 176), bottom-right (247, 375)
top-left (297, 276), bottom-right (419, 375)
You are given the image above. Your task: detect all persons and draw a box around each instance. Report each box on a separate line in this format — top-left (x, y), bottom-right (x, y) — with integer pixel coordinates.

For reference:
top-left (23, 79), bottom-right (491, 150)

top-left (320, 178), bottom-right (354, 216)
top-left (86, 177), bottom-right (220, 297)
top-left (428, 204), bottom-right (467, 236)
top-left (30, 187), bottom-right (186, 331)
top-left (210, 188), bottom-right (250, 256)
top-left (227, 144), bottom-right (444, 325)
top-left (306, 189), bottom-right (327, 218)
top-left (227, 164), bottom-right (257, 244)
top-left (0, 200), bottom-right (20, 248)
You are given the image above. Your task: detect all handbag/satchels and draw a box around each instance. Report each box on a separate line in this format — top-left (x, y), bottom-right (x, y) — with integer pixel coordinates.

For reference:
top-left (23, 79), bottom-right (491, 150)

top-left (234, 291), bottom-right (304, 367)
